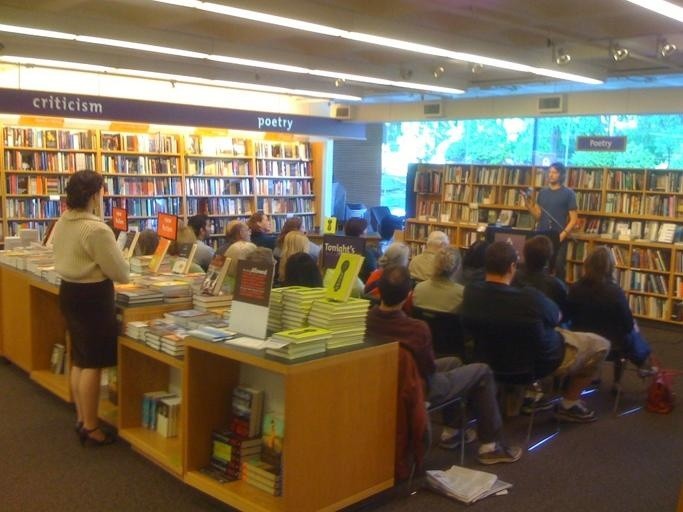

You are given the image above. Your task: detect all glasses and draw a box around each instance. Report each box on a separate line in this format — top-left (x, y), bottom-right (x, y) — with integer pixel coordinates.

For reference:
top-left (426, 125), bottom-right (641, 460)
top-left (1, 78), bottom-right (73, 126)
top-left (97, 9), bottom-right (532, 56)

top-left (239, 227), bottom-right (250, 232)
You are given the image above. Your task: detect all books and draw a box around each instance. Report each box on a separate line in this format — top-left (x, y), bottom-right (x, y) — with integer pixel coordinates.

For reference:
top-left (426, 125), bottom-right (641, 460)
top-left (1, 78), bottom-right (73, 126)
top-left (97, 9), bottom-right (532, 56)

top-left (1, 128), bottom-right (315, 243)
top-left (562, 167), bottom-right (683, 324)
top-left (407, 163), bottom-right (549, 262)
top-left (1, 229), bottom-right (370, 496)
top-left (424, 463), bottom-right (514, 504)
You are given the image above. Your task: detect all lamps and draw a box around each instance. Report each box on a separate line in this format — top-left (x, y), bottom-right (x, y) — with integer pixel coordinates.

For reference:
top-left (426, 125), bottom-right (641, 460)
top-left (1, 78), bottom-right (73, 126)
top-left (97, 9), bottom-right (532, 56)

top-left (542, 30), bottom-right (675, 65)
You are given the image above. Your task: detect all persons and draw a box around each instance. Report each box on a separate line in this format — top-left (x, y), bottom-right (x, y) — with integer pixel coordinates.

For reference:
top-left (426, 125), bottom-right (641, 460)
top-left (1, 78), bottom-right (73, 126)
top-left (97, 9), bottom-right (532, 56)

top-left (107, 211), bottom-right (370, 300)
top-left (520, 162), bottom-right (578, 282)
top-left (364, 214), bottom-right (658, 465)
top-left (52, 169), bottom-right (130, 446)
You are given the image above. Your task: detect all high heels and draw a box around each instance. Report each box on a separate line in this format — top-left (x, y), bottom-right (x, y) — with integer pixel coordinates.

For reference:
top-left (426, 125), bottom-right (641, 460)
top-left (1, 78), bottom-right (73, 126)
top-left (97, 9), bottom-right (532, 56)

top-left (637, 365), bottom-right (661, 385)
top-left (74, 421), bottom-right (115, 437)
top-left (611, 382), bottom-right (625, 397)
top-left (80, 427), bottom-right (115, 447)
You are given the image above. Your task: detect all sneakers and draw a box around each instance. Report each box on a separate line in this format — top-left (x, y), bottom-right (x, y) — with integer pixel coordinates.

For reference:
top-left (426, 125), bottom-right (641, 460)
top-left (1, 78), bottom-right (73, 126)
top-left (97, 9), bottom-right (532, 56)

top-left (476, 442), bottom-right (521, 465)
top-left (558, 401), bottom-right (598, 424)
top-left (519, 392), bottom-right (555, 415)
top-left (441, 428), bottom-right (476, 450)
